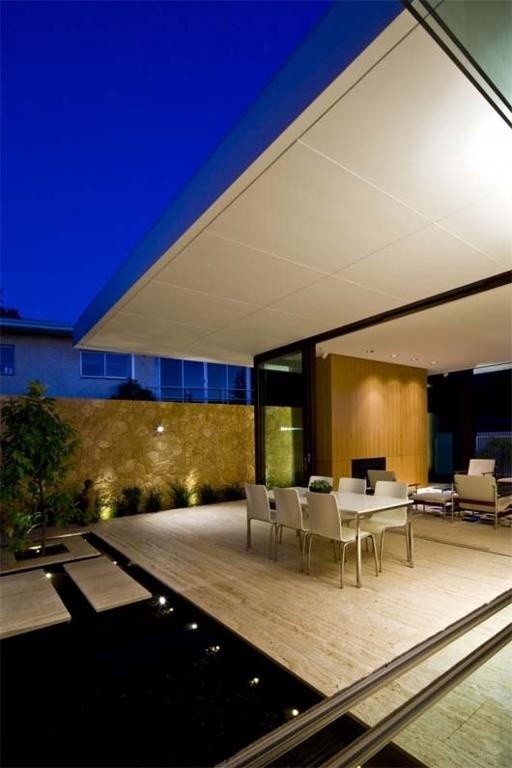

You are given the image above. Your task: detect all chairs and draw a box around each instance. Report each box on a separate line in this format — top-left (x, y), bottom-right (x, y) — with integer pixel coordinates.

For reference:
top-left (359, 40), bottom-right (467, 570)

top-left (240, 457), bottom-right (511, 590)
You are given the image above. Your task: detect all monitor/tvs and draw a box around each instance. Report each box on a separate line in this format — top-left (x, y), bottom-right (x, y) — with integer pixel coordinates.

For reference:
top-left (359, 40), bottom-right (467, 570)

top-left (352, 456), bottom-right (387, 487)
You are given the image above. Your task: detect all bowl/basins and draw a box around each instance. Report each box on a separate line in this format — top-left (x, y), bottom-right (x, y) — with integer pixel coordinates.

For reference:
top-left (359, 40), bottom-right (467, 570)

top-left (308, 485), bottom-right (334, 493)
